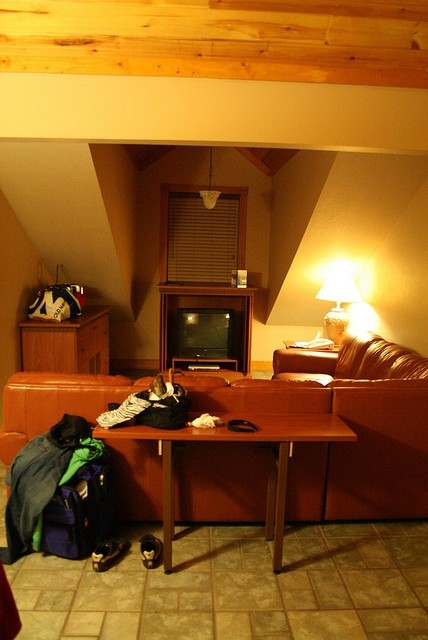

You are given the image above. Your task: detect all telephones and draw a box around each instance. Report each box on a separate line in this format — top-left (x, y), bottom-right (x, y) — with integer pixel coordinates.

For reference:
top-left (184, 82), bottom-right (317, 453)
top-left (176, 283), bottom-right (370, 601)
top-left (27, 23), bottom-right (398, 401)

top-left (304, 338), bottom-right (335, 349)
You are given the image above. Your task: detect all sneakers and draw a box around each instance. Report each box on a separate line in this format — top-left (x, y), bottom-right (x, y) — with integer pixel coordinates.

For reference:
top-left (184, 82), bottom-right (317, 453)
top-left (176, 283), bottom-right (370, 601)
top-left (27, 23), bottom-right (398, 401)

top-left (139, 534), bottom-right (164, 570)
top-left (91, 536), bottom-right (131, 572)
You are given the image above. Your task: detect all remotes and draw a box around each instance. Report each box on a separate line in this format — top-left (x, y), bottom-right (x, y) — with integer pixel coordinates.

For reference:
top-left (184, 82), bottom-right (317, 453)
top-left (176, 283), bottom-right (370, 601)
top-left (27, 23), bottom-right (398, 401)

top-left (165, 282), bottom-right (183, 287)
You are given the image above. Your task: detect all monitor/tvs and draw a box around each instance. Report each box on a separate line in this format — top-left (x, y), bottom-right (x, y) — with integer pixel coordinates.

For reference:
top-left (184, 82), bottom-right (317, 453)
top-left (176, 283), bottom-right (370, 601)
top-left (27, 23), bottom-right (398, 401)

top-left (176, 308), bottom-right (235, 358)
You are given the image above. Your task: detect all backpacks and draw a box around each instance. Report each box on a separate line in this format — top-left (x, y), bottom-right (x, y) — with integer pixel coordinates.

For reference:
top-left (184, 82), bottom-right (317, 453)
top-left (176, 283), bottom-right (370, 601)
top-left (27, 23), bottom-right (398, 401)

top-left (39, 457), bottom-right (110, 561)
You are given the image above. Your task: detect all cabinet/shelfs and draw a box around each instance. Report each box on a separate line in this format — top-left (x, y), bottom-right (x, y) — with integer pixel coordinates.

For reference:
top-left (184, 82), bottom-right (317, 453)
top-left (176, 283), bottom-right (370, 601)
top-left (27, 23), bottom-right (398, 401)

top-left (18, 305), bottom-right (112, 375)
top-left (157, 281), bottom-right (258, 375)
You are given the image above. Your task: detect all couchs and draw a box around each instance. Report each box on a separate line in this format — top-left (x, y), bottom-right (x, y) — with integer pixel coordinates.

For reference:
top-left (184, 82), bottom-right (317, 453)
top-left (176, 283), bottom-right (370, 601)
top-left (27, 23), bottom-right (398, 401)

top-left (0, 330), bottom-right (427, 522)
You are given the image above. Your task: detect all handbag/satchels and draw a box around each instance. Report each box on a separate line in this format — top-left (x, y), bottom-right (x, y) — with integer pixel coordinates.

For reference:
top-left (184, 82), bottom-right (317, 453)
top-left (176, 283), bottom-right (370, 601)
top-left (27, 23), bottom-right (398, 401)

top-left (27, 285), bottom-right (82, 323)
top-left (130, 389), bottom-right (191, 431)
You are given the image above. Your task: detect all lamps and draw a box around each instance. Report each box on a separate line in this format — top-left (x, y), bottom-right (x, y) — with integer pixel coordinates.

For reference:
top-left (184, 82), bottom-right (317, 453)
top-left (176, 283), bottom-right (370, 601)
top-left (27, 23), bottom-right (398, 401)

top-left (199, 146), bottom-right (221, 209)
top-left (315, 274), bottom-right (361, 347)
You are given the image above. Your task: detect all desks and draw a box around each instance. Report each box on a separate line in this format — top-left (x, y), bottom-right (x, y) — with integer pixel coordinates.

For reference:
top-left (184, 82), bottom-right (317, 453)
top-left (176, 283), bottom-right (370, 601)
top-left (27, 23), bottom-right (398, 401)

top-left (92, 414), bottom-right (358, 573)
top-left (283, 340), bottom-right (342, 353)
